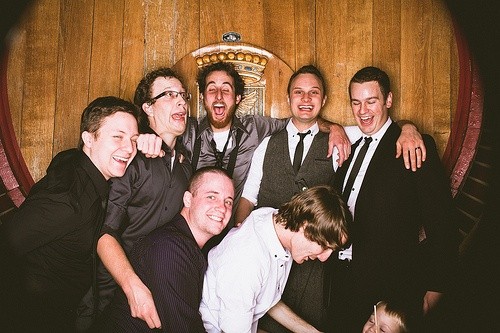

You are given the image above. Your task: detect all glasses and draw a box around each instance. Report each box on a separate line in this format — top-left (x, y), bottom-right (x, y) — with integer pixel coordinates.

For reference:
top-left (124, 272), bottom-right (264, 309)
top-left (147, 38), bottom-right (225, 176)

top-left (147, 91), bottom-right (192, 106)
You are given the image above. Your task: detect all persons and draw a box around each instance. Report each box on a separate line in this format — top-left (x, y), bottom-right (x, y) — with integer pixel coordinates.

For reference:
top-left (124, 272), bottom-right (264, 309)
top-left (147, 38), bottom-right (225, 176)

top-left (76, 68), bottom-right (193, 333)
top-left (193, 62), bottom-right (351, 212)
top-left (199, 185), bottom-right (352, 333)
top-left (0, 96), bottom-right (163, 333)
top-left (94, 166), bottom-right (234, 333)
top-left (321, 66), bottom-right (457, 333)
top-left (236, 64), bottom-right (426, 333)
top-left (363, 301), bottom-right (408, 333)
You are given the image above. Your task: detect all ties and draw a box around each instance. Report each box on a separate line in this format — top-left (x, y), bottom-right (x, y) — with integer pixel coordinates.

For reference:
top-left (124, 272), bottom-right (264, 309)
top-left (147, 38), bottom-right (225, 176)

top-left (343, 137), bottom-right (373, 203)
top-left (293, 130), bottom-right (311, 174)
top-left (211, 131), bottom-right (232, 168)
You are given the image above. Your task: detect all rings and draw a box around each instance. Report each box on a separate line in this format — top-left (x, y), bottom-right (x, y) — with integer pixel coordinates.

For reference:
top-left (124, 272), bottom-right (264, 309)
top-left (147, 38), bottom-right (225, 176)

top-left (415, 147), bottom-right (420, 150)
